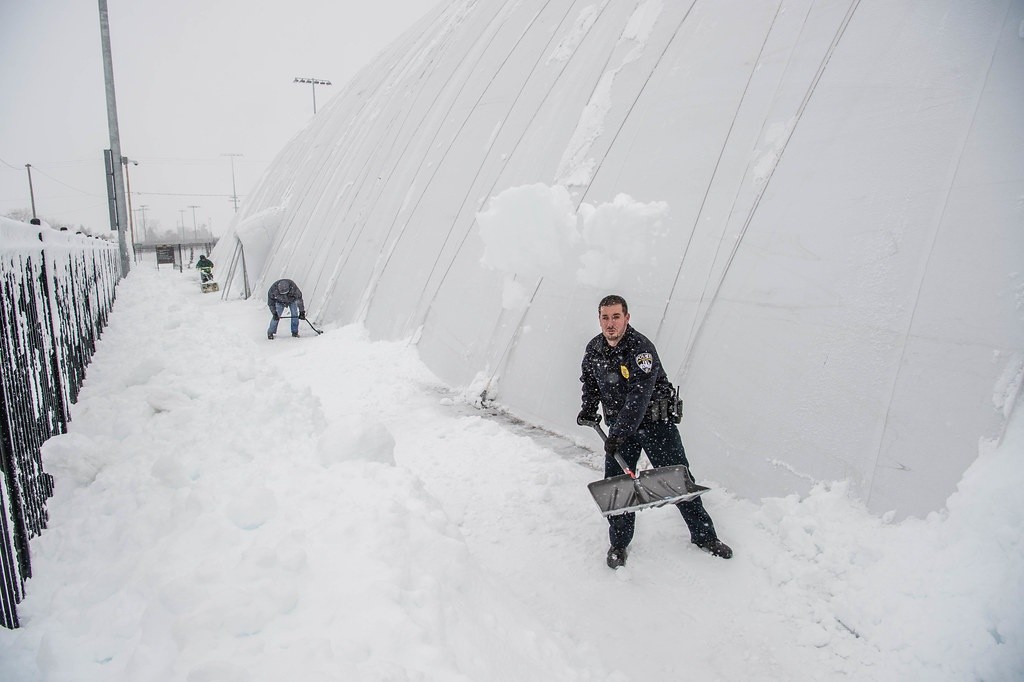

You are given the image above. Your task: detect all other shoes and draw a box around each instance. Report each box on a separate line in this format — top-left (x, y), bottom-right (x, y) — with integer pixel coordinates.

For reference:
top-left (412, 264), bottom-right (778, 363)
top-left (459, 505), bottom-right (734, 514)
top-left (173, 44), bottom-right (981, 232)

top-left (268, 333), bottom-right (273, 339)
top-left (292, 332), bottom-right (300, 338)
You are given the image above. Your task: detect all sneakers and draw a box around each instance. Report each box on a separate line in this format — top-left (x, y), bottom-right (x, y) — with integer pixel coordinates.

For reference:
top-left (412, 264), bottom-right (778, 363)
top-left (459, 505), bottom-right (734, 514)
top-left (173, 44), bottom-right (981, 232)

top-left (699, 538), bottom-right (732, 558)
top-left (607, 546), bottom-right (628, 569)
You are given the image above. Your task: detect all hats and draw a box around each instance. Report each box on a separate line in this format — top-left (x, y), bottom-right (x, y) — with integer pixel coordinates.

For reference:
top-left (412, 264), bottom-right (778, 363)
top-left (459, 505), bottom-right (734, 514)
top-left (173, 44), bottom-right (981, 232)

top-left (278, 281), bottom-right (289, 294)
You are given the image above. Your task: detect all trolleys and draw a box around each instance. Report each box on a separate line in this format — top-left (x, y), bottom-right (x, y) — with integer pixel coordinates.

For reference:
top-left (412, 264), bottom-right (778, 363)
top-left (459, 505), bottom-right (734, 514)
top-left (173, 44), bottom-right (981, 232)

top-left (196, 266), bottom-right (220, 293)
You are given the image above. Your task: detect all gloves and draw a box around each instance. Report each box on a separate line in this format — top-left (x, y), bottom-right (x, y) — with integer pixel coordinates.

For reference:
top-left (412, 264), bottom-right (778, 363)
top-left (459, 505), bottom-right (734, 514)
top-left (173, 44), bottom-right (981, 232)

top-left (273, 311), bottom-right (279, 321)
top-left (577, 411), bottom-right (595, 426)
top-left (299, 311), bottom-right (305, 320)
top-left (604, 439), bottom-right (618, 458)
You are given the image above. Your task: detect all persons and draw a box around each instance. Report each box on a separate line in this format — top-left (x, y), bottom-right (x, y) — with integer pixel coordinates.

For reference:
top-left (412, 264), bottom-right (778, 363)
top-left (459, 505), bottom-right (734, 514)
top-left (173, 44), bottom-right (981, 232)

top-left (195, 255), bottom-right (214, 289)
top-left (576, 294), bottom-right (733, 569)
top-left (266, 278), bottom-right (306, 340)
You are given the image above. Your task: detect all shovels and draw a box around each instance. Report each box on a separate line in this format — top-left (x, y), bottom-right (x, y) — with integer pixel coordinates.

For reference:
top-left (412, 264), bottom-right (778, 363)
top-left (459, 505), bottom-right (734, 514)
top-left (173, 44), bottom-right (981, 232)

top-left (582, 417), bottom-right (713, 518)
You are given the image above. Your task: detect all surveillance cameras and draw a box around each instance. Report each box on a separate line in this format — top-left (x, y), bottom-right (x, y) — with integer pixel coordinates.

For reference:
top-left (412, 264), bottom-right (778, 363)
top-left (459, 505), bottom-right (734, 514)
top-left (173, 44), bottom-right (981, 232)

top-left (133, 161), bottom-right (138, 165)
